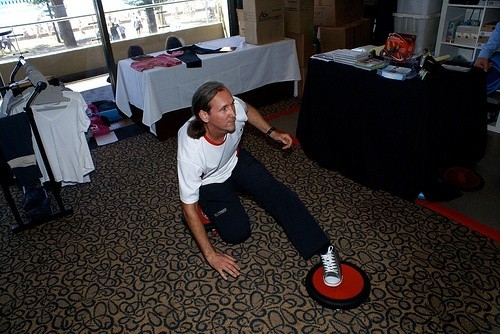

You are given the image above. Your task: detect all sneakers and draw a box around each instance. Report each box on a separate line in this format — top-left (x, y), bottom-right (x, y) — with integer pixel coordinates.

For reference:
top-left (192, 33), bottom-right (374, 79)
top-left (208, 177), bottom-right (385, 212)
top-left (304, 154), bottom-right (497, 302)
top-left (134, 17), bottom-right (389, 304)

top-left (320, 245), bottom-right (343, 287)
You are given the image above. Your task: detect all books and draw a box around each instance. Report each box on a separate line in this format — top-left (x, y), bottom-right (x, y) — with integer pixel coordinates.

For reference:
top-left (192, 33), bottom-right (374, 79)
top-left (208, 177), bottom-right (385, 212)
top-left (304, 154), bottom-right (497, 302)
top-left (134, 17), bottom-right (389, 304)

top-left (311, 44), bottom-right (411, 81)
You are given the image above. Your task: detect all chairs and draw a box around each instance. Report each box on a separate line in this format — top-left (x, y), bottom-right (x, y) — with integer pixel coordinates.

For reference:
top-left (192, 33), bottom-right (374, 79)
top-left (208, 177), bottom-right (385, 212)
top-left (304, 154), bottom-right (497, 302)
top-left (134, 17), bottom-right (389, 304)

top-left (128, 46), bottom-right (143, 57)
top-left (165, 35), bottom-right (183, 51)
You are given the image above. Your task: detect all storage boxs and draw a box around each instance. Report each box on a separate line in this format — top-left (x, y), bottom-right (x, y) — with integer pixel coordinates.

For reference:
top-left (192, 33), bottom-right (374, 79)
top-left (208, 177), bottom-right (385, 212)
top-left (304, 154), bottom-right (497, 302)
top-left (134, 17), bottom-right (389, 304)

top-left (388, 0), bottom-right (443, 57)
top-left (236, 0), bottom-right (373, 92)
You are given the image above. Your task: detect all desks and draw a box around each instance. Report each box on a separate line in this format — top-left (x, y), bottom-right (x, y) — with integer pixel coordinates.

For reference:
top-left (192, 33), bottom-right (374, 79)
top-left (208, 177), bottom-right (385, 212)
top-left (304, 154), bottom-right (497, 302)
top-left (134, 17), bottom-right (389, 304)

top-left (0, 89), bottom-right (78, 180)
top-left (309, 44), bottom-right (486, 199)
top-left (118, 33), bottom-right (297, 143)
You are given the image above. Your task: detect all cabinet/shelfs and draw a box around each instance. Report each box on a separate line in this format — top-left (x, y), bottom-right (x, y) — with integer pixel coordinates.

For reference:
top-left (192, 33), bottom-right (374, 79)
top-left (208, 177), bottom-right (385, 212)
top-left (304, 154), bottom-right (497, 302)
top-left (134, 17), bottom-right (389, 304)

top-left (436, 0), bottom-right (500, 135)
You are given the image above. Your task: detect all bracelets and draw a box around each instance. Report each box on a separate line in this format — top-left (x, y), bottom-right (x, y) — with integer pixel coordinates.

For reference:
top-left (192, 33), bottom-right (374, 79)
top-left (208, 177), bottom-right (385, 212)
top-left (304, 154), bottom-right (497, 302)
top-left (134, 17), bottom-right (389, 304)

top-left (266, 127), bottom-right (275, 137)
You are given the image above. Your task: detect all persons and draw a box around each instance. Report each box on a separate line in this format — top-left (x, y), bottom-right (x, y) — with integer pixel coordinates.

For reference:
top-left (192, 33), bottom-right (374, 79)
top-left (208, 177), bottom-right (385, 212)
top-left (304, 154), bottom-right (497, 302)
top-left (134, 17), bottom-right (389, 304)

top-left (109, 21), bottom-right (126, 42)
top-left (133, 16), bottom-right (143, 35)
top-left (176, 81), bottom-right (344, 287)
top-left (475, 21), bottom-right (500, 94)
